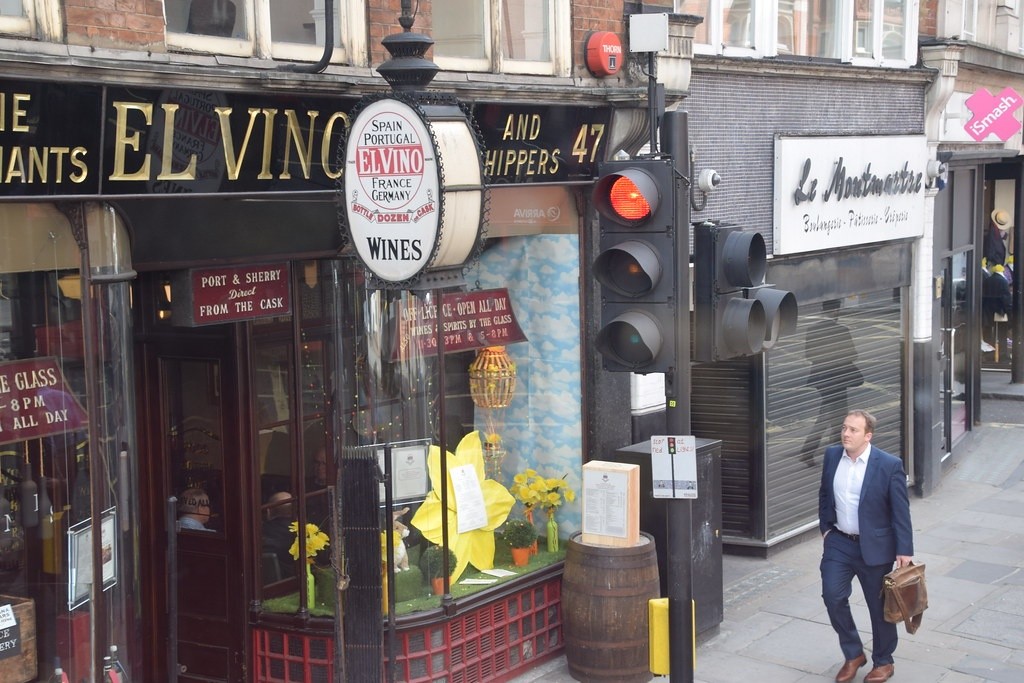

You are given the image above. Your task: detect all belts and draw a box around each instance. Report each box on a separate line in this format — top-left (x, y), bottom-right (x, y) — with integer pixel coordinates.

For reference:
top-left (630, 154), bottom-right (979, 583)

top-left (834, 527), bottom-right (859, 542)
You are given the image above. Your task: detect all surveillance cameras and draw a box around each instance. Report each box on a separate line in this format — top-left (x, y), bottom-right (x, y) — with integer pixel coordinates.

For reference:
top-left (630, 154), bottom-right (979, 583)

top-left (927, 160), bottom-right (946, 177)
top-left (698, 169), bottom-right (721, 191)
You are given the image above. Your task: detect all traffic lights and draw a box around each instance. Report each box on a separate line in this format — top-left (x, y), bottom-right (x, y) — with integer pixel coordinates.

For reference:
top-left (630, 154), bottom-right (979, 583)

top-left (693, 219), bottom-right (799, 367)
top-left (591, 158), bottom-right (677, 375)
top-left (668, 437), bottom-right (677, 455)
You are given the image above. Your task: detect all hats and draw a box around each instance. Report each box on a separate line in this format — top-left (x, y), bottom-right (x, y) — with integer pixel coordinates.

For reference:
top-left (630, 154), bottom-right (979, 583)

top-left (821, 298), bottom-right (842, 311)
top-left (990, 208), bottom-right (1012, 230)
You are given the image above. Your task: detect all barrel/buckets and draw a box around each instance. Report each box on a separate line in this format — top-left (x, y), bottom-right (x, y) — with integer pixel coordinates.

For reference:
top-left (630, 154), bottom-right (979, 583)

top-left (562, 531), bottom-right (660, 683)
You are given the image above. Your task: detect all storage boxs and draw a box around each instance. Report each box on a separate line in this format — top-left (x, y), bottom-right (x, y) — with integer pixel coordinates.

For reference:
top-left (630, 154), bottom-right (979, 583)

top-left (580, 458), bottom-right (640, 549)
top-left (0, 595), bottom-right (37, 683)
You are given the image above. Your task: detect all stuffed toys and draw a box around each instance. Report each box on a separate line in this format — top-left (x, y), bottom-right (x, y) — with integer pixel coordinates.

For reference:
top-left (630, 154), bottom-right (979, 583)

top-left (386, 506), bottom-right (411, 572)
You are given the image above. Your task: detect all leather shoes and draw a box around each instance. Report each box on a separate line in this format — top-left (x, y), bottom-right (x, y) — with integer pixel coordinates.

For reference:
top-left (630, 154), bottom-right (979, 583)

top-left (864, 663), bottom-right (894, 683)
top-left (836, 653), bottom-right (868, 683)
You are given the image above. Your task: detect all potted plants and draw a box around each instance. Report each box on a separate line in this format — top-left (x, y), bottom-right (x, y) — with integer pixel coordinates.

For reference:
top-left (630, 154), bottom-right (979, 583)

top-left (420, 545), bottom-right (456, 594)
top-left (503, 519), bottom-right (540, 566)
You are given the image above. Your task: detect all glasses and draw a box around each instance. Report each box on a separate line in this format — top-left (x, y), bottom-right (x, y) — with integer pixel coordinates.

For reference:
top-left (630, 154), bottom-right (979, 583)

top-left (201, 502), bottom-right (213, 508)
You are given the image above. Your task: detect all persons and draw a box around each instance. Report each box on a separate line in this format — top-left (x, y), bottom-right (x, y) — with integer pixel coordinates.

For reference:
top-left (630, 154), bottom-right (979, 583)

top-left (799, 300), bottom-right (865, 467)
top-left (174, 442), bottom-right (328, 582)
top-left (817, 408), bottom-right (914, 683)
top-left (979, 207), bottom-right (1014, 365)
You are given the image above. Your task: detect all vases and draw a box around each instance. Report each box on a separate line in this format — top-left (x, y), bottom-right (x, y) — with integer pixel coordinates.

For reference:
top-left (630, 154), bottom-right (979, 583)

top-left (305, 562), bottom-right (315, 610)
top-left (524, 510), bottom-right (538, 555)
top-left (381, 565), bottom-right (388, 616)
top-left (547, 513), bottom-right (559, 553)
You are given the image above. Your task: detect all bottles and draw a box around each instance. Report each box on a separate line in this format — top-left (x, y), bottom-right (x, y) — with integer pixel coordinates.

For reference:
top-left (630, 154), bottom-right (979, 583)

top-left (104, 657), bottom-right (112, 683)
top-left (111, 646), bottom-right (123, 683)
top-left (37, 478), bottom-right (54, 539)
top-left (55, 669), bottom-right (62, 683)
top-left (20, 463), bottom-right (38, 527)
top-left (0, 483), bottom-right (11, 549)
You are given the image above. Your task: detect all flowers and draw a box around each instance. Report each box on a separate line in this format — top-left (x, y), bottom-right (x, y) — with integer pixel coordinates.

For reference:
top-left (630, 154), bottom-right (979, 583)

top-left (288, 523), bottom-right (327, 559)
top-left (512, 469), bottom-right (546, 509)
top-left (381, 532), bottom-right (400, 561)
top-left (546, 478), bottom-right (574, 512)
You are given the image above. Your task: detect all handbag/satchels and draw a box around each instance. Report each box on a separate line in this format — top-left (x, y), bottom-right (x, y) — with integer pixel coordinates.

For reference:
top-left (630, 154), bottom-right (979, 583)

top-left (835, 359), bottom-right (864, 391)
top-left (984, 219), bottom-right (1006, 267)
top-left (883, 561), bottom-right (929, 635)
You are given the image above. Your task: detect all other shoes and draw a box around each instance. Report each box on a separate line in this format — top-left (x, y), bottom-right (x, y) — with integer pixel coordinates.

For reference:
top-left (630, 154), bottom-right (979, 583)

top-left (799, 452), bottom-right (820, 467)
